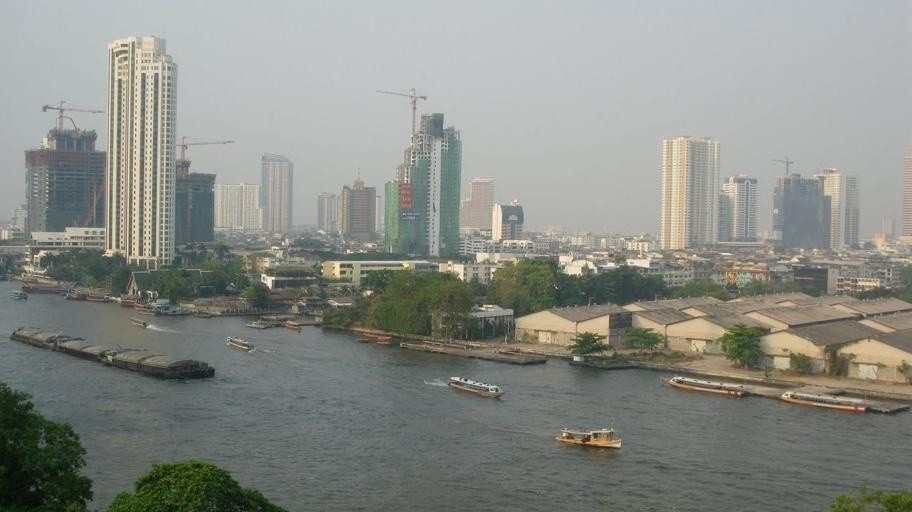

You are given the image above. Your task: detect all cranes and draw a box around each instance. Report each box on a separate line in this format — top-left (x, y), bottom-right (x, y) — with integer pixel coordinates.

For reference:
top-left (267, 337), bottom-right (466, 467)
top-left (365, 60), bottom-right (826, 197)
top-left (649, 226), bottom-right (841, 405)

top-left (41, 98), bottom-right (108, 134)
top-left (769, 154), bottom-right (795, 177)
top-left (176, 134), bottom-right (238, 159)
top-left (374, 86), bottom-right (429, 149)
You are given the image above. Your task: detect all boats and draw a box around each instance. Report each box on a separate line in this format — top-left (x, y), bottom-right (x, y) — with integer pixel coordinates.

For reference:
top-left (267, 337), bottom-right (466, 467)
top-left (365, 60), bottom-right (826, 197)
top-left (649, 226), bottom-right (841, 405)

top-left (656, 375), bottom-right (745, 398)
top-left (190, 311), bottom-right (212, 319)
top-left (448, 376), bottom-right (506, 399)
top-left (554, 425), bottom-right (626, 451)
top-left (780, 391), bottom-right (871, 415)
top-left (224, 315), bottom-right (303, 354)
top-left (374, 335), bottom-right (394, 346)
top-left (0, 274), bottom-right (184, 328)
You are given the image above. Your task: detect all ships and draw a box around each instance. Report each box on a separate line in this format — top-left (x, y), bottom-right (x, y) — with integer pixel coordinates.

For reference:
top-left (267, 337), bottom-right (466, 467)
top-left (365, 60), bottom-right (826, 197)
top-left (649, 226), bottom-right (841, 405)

top-left (5, 324), bottom-right (217, 382)
